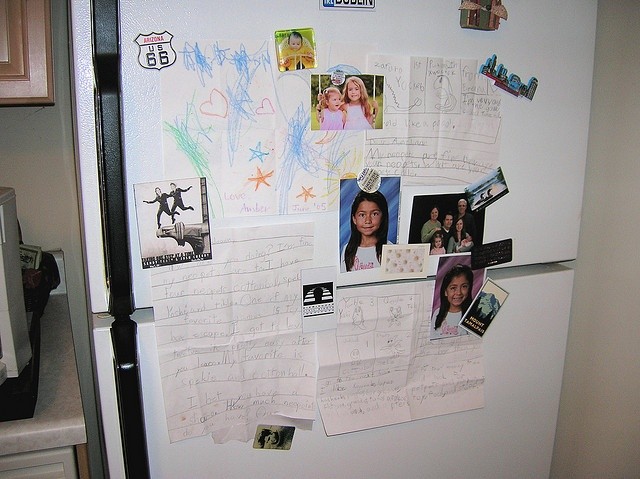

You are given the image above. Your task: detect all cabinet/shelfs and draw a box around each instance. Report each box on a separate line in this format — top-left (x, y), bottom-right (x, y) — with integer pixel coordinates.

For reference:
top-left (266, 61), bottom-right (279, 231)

top-left (3, 445), bottom-right (79, 475)
top-left (0, 0), bottom-right (53, 105)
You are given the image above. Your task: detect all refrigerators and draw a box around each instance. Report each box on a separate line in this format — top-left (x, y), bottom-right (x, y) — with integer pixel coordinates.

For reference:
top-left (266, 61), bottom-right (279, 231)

top-left (66, 1), bottom-right (602, 479)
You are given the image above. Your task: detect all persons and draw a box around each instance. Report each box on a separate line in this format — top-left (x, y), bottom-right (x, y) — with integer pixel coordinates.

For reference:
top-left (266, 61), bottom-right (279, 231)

top-left (431, 233), bottom-right (446, 255)
top-left (317, 75), bottom-right (379, 130)
top-left (280, 32), bottom-right (315, 70)
top-left (455, 234), bottom-right (473, 252)
top-left (447, 219), bottom-right (475, 253)
top-left (142, 187), bottom-right (180, 229)
top-left (420, 205), bottom-right (441, 242)
top-left (317, 88), bottom-right (347, 130)
top-left (454, 199), bottom-right (477, 236)
top-left (167, 183), bottom-right (194, 224)
top-left (257, 427), bottom-right (295, 450)
top-left (340, 189), bottom-right (394, 271)
top-left (428, 213), bottom-right (453, 250)
top-left (432, 264), bottom-right (474, 338)
